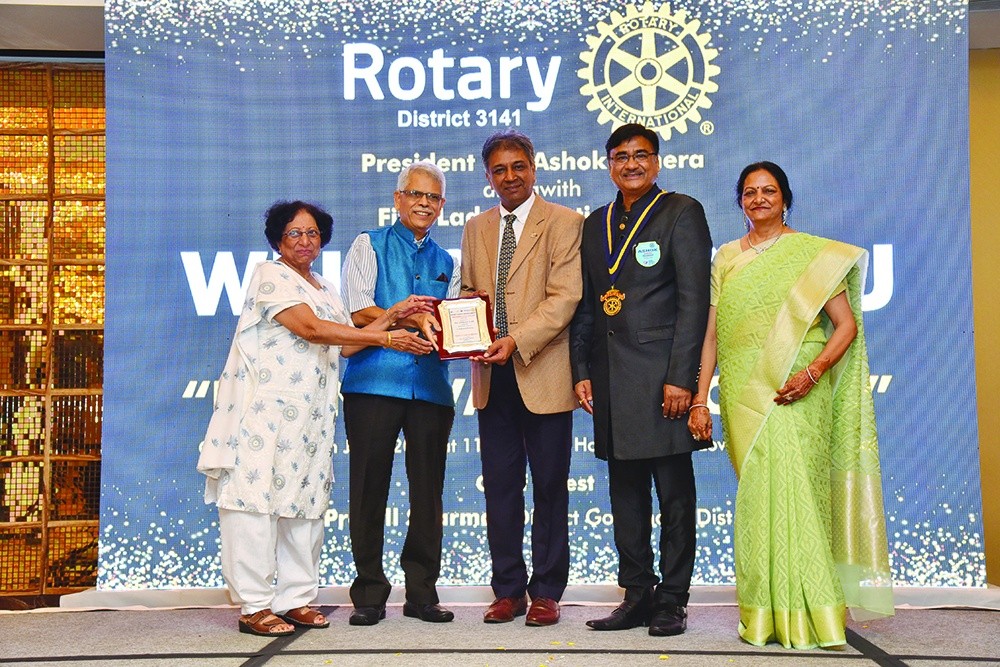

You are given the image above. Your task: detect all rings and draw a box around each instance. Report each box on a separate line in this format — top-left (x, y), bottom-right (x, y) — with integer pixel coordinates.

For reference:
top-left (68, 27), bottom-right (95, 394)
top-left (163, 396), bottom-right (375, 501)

top-left (579, 399), bottom-right (584, 405)
top-left (787, 397), bottom-right (792, 400)
top-left (693, 435), bottom-right (699, 441)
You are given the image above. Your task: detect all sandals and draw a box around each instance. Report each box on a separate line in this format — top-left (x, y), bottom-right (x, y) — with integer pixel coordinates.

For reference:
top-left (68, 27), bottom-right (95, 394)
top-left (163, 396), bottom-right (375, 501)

top-left (239, 608), bottom-right (295, 635)
top-left (275, 606), bottom-right (330, 627)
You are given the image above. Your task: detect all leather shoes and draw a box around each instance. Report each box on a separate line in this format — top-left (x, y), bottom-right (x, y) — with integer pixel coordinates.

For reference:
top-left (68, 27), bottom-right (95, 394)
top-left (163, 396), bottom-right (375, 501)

top-left (649, 607), bottom-right (688, 636)
top-left (349, 608), bottom-right (386, 625)
top-left (484, 596), bottom-right (527, 622)
top-left (525, 598), bottom-right (560, 625)
top-left (402, 602), bottom-right (454, 622)
top-left (586, 605), bottom-right (652, 630)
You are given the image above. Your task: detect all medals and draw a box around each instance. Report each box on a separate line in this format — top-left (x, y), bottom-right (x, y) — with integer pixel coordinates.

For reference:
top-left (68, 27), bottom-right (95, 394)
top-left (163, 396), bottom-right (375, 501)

top-left (602, 297), bottom-right (622, 316)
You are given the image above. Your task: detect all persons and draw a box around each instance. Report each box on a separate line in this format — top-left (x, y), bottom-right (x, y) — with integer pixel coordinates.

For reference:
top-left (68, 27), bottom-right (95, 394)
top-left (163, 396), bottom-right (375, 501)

top-left (569, 124), bottom-right (714, 636)
top-left (339, 161), bottom-right (461, 625)
top-left (458, 129), bottom-right (585, 625)
top-left (687, 160), bottom-right (895, 650)
top-left (197, 201), bottom-right (433, 636)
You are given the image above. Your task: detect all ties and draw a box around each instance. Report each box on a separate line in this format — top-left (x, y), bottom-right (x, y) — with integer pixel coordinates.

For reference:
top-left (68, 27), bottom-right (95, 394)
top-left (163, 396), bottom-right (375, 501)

top-left (496, 215), bottom-right (517, 338)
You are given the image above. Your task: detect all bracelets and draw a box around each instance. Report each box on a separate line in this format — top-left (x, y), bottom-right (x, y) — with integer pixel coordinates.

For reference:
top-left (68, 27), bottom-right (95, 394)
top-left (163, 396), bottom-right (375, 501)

top-left (689, 404), bottom-right (709, 414)
top-left (806, 368), bottom-right (818, 384)
top-left (383, 332), bottom-right (392, 349)
top-left (387, 308), bottom-right (396, 326)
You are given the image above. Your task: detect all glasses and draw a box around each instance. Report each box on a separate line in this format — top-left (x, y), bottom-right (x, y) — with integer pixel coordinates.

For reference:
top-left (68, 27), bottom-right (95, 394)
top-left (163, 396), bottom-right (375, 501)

top-left (607, 153), bottom-right (657, 162)
top-left (400, 189), bottom-right (443, 202)
top-left (281, 230), bottom-right (324, 239)
